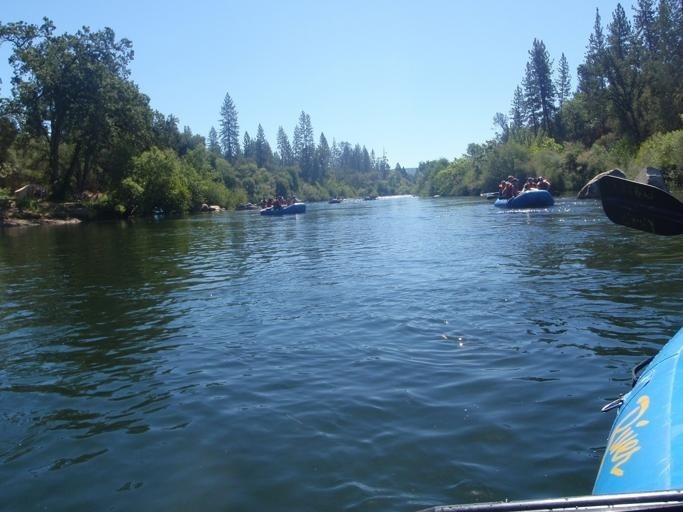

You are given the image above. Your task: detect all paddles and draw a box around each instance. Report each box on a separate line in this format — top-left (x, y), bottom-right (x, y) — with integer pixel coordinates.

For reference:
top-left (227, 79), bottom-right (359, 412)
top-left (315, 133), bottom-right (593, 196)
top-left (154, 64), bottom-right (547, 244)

top-left (598, 175), bottom-right (682, 237)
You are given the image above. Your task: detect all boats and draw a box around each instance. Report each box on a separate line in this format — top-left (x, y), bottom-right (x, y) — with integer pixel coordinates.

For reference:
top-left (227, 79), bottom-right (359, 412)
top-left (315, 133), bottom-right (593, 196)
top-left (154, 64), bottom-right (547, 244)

top-left (492, 187), bottom-right (554, 208)
top-left (593, 314), bottom-right (683, 496)
top-left (144, 193), bottom-right (374, 218)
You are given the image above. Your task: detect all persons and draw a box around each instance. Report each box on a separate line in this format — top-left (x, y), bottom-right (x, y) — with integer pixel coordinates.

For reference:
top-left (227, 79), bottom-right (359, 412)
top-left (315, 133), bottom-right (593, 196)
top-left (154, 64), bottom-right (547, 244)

top-left (266, 194), bottom-right (301, 211)
top-left (497, 175), bottom-right (551, 199)
